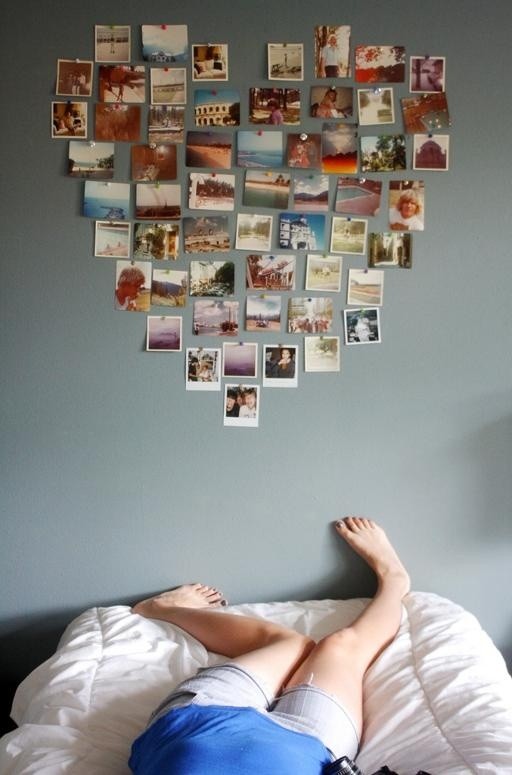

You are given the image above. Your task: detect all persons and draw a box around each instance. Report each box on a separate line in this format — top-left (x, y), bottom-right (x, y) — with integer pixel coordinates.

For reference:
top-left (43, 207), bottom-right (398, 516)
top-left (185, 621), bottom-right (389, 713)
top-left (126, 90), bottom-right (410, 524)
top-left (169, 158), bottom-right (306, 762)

top-left (188, 357), bottom-right (197, 382)
top-left (321, 34), bottom-right (339, 79)
top-left (390, 190), bottom-right (423, 231)
top-left (199, 365), bottom-right (213, 382)
top-left (239, 389), bottom-right (257, 418)
top-left (237, 389), bottom-right (243, 406)
top-left (317, 89), bottom-right (344, 119)
top-left (62, 111), bottom-right (73, 130)
top-left (227, 390), bottom-right (239, 417)
top-left (266, 100), bottom-right (284, 124)
top-left (127, 516), bottom-right (411, 770)
top-left (66, 72), bottom-right (87, 96)
top-left (271, 349), bottom-right (295, 378)
top-left (110, 32), bottom-right (115, 55)
top-left (116, 268), bottom-right (145, 312)
top-left (428, 61), bottom-right (443, 91)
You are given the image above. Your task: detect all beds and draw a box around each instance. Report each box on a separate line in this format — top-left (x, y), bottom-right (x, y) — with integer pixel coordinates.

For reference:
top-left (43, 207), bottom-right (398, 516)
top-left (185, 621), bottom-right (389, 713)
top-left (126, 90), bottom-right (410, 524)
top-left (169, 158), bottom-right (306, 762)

top-left (1, 591), bottom-right (511, 775)
